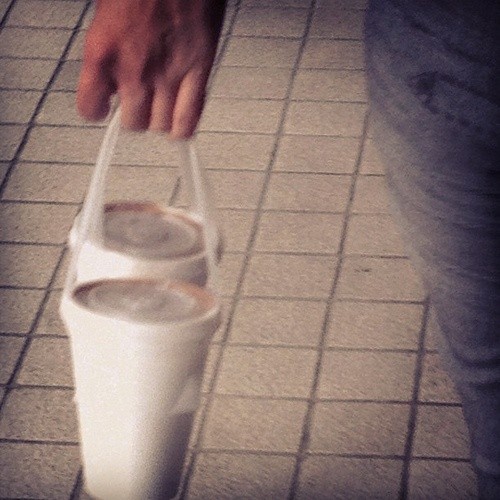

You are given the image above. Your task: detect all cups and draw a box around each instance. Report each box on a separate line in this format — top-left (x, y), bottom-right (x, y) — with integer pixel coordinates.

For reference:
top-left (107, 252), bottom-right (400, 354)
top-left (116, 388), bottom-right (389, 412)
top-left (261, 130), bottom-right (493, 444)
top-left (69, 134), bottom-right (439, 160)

top-left (67, 201), bottom-right (223, 287)
top-left (60, 278), bottom-right (224, 500)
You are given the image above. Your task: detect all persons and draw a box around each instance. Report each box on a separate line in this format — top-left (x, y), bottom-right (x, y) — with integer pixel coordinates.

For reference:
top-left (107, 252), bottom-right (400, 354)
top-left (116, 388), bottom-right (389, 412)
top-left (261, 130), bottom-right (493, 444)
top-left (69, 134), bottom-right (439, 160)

top-left (74, 0), bottom-right (499, 499)
